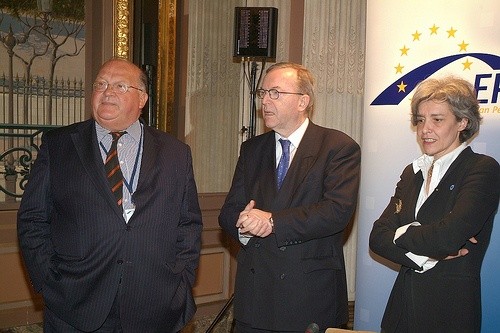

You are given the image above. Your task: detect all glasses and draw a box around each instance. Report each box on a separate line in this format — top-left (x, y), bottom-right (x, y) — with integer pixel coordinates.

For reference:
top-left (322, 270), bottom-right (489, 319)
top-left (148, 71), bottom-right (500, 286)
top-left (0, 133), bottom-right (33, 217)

top-left (93, 80), bottom-right (144, 95)
top-left (256, 88), bottom-right (305, 100)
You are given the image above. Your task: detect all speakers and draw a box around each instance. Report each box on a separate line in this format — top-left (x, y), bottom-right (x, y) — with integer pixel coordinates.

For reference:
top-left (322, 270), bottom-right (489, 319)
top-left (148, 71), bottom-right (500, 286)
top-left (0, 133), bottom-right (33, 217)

top-left (234, 7), bottom-right (278, 57)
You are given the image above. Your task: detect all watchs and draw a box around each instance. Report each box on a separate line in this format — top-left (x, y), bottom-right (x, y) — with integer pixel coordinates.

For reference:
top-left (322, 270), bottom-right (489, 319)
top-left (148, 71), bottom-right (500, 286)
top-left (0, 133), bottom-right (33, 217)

top-left (269, 213), bottom-right (275, 232)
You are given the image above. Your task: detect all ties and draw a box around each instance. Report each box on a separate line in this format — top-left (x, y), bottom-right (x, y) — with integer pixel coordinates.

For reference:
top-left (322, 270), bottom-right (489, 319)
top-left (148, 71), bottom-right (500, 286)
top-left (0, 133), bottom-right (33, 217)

top-left (276, 137), bottom-right (290, 190)
top-left (104, 131), bottom-right (127, 213)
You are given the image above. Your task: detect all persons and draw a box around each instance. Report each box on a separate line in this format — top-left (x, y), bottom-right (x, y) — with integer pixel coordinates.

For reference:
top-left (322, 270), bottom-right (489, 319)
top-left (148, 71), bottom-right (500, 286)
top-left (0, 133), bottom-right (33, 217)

top-left (16, 58), bottom-right (204, 333)
top-left (368, 77), bottom-right (500, 333)
top-left (216, 62), bottom-right (363, 333)
